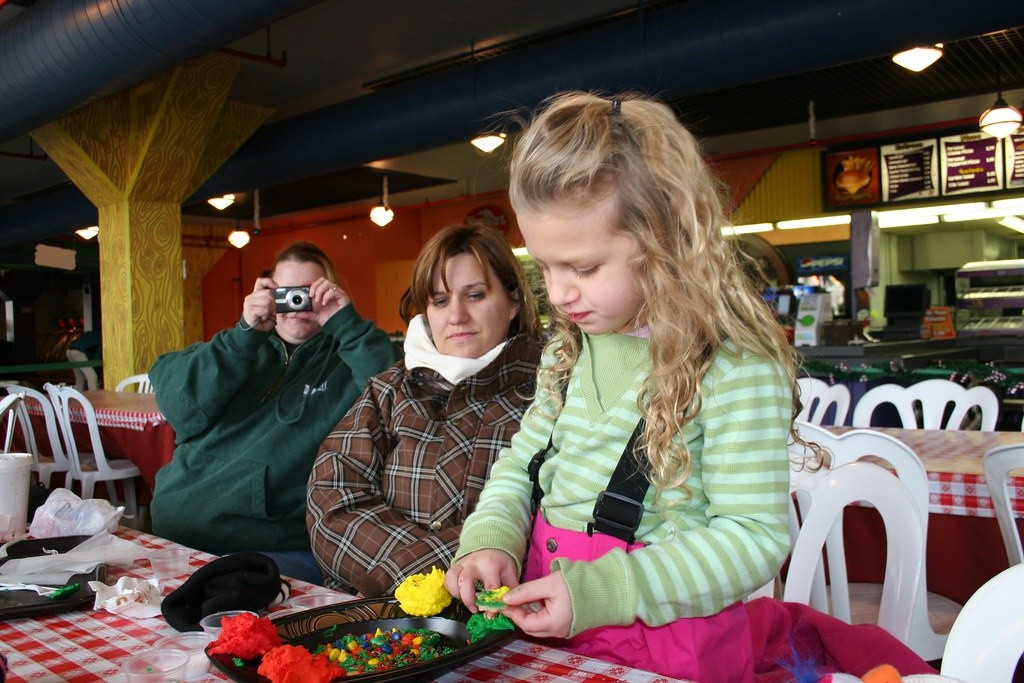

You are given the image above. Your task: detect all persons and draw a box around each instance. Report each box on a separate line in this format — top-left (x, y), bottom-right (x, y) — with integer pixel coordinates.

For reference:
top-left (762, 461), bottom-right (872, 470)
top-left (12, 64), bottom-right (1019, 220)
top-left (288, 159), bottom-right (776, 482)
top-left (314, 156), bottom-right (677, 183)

top-left (306, 222), bottom-right (549, 597)
top-left (66, 330), bottom-right (102, 391)
top-left (149, 241), bottom-right (405, 579)
top-left (445, 89), bottom-right (812, 640)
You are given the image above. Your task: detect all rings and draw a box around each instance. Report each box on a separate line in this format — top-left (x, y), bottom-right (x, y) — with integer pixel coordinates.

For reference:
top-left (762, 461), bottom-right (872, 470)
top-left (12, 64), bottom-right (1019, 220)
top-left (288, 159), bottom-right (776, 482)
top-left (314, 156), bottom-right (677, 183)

top-left (334, 284), bottom-right (337, 287)
top-left (333, 287), bottom-right (337, 291)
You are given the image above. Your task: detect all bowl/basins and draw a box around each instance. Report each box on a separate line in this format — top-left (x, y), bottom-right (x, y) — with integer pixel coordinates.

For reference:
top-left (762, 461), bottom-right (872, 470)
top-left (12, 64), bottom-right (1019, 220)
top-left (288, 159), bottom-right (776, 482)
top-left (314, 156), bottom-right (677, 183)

top-left (152, 632), bottom-right (216, 677)
top-left (146, 549), bottom-right (190, 577)
top-left (119, 649), bottom-right (189, 683)
top-left (200, 610), bottom-right (259, 638)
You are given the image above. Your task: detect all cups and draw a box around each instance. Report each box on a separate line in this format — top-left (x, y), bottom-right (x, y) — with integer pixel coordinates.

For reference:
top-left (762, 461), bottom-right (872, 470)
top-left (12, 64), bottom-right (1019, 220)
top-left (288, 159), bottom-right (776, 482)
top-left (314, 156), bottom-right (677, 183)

top-left (0, 453), bottom-right (34, 544)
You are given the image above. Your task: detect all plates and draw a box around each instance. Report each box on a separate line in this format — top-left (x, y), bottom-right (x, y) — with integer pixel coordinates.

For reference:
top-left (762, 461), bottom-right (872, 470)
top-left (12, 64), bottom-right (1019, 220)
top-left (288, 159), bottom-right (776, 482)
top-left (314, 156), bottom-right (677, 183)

top-left (205, 583), bottom-right (530, 683)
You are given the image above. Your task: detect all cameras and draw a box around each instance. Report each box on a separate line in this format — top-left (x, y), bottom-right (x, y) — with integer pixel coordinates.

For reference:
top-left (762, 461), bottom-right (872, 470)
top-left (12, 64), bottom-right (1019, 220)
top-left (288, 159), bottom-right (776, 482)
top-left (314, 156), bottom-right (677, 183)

top-left (272, 286), bottom-right (313, 314)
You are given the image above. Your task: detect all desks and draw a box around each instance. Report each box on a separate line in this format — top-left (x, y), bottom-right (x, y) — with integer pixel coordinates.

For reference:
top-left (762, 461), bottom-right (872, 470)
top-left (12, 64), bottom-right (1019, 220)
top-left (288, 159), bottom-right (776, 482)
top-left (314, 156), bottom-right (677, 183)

top-left (778, 425), bottom-right (1024, 606)
top-left (0, 523), bottom-right (697, 683)
top-left (0, 389), bottom-right (177, 530)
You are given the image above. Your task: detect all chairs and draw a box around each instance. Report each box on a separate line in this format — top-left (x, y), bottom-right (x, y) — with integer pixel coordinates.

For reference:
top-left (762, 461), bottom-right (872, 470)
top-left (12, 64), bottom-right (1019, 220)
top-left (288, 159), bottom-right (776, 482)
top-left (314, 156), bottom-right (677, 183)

top-left (744, 375), bottom-right (1024, 683)
top-left (0, 372), bottom-right (155, 531)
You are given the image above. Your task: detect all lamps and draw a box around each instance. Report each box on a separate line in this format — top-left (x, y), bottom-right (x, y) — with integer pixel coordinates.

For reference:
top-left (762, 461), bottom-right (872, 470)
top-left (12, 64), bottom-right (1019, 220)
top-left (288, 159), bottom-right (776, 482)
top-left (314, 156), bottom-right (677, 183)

top-left (369, 176), bottom-right (394, 226)
top-left (892, 44), bottom-right (945, 72)
top-left (979, 69), bottom-right (1023, 139)
top-left (228, 209), bottom-right (249, 249)
top-left (471, 39), bottom-right (505, 155)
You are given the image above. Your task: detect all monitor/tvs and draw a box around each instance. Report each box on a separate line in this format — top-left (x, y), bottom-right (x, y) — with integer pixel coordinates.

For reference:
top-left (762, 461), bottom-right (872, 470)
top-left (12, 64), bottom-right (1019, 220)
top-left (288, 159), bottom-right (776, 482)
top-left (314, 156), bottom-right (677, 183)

top-left (885, 283), bottom-right (931, 318)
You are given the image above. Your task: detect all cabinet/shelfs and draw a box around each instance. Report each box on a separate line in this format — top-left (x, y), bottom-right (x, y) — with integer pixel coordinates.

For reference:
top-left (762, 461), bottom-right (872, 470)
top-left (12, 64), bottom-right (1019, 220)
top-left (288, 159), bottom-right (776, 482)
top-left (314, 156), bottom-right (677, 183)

top-left (955, 257), bottom-right (1024, 338)
